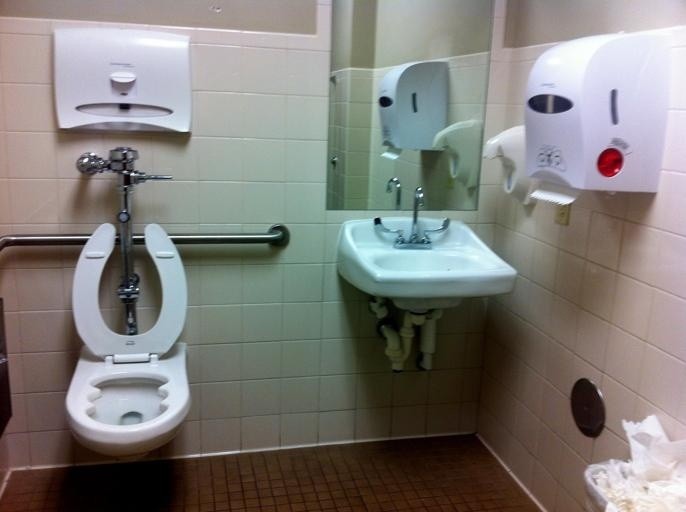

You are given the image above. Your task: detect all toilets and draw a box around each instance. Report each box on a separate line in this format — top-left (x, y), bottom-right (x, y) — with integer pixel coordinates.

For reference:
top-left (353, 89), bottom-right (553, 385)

top-left (64, 222), bottom-right (192, 457)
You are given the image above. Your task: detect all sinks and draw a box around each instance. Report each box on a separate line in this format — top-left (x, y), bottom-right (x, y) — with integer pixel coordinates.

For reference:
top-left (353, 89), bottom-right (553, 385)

top-left (336, 217), bottom-right (518, 298)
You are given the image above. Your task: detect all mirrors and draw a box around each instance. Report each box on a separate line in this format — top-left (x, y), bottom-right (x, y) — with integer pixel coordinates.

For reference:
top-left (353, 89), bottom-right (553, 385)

top-left (324, 0), bottom-right (495, 214)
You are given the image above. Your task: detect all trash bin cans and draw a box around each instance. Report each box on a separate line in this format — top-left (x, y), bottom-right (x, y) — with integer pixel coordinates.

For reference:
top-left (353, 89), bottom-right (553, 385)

top-left (583, 459), bottom-right (686, 512)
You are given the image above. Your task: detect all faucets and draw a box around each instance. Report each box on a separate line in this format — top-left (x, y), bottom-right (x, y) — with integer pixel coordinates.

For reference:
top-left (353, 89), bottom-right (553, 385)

top-left (408, 186), bottom-right (425, 242)
top-left (386, 177), bottom-right (401, 210)
